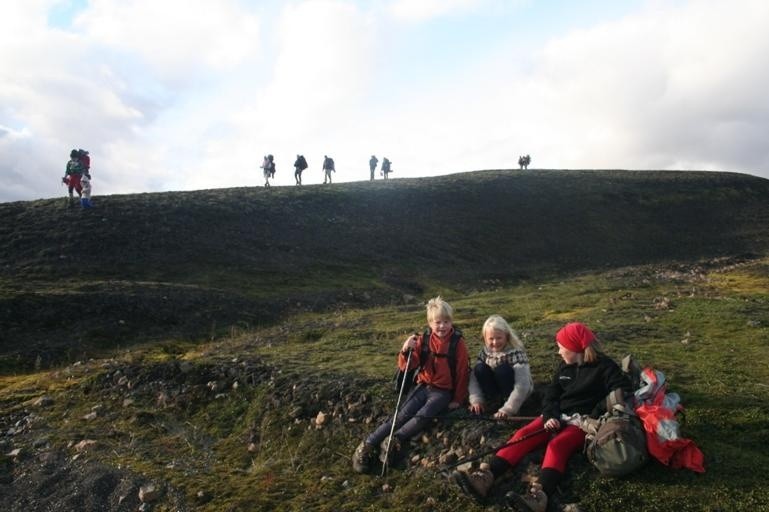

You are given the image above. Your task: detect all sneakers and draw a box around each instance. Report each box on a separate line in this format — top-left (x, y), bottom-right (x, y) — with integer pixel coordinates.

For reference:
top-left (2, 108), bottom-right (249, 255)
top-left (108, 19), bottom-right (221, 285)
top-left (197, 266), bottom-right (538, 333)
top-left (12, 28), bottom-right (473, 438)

top-left (352, 442), bottom-right (377, 473)
top-left (379, 435), bottom-right (401, 467)
top-left (451, 468), bottom-right (496, 506)
top-left (504, 490), bottom-right (548, 512)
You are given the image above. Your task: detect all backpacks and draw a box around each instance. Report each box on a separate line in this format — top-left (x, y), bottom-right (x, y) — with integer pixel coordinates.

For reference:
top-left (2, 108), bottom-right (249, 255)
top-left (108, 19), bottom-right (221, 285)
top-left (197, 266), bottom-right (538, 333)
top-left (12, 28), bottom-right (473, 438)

top-left (392, 328), bottom-right (461, 393)
top-left (582, 388), bottom-right (649, 478)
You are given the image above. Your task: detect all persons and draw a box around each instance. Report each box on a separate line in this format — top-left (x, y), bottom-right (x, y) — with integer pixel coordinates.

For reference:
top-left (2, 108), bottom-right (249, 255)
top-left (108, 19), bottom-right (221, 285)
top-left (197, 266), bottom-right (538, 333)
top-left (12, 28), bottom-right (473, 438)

top-left (260, 156), bottom-right (272, 189)
top-left (323, 155), bottom-right (335, 184)
top-left (80, 175), bottom-right (91, 211)
top-left (370, 155), bottom-right (378, 182)
top-left (381, 157), bottom-right (390, 180)
top-left (468, 314), bottom-right (534, 419)
top-left (294, 155), bottom-right (302, 186)
top-left (518, 155), bottom-right (528, 169)
top-left (351, 295), bottom-right (468, 472)
top-left (63, 149), bottom-right (82, 208)
top-left (453, 322), bottom-right (634, 512)
